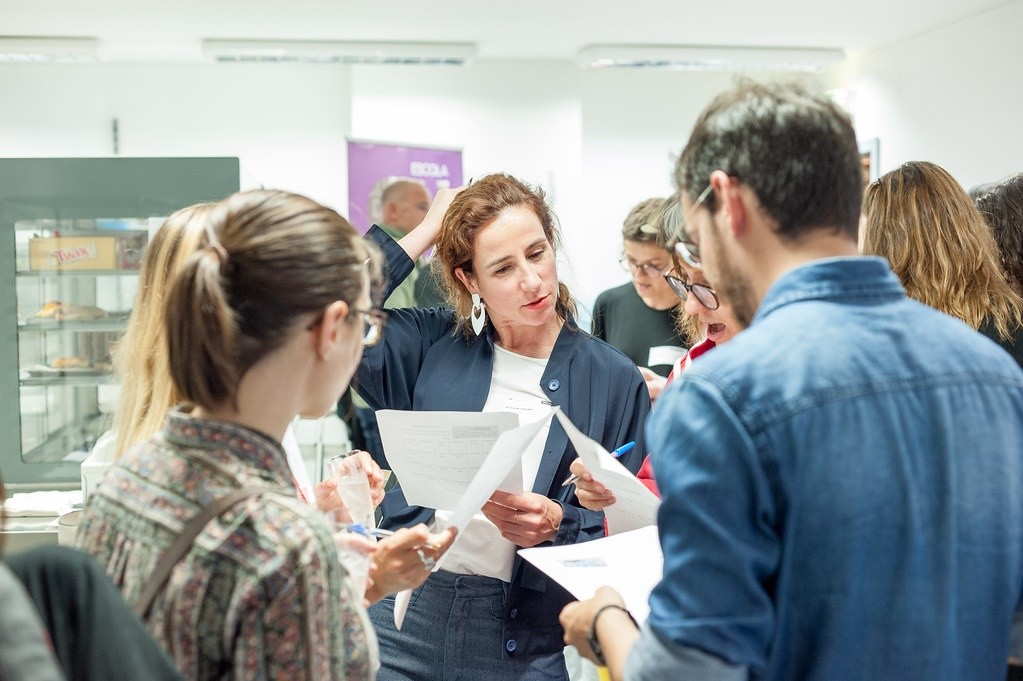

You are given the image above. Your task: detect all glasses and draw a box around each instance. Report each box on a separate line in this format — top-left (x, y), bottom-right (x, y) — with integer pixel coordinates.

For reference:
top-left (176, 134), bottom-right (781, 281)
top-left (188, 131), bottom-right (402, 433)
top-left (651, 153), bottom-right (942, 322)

top-left (662, 265), bottom-right (720, 311)
top-left (307, 307), bottom-right (388, 347)
top-left (619, 249), bottom-right (670, 278)
top-left (664, 176), bottom-right (738, 281)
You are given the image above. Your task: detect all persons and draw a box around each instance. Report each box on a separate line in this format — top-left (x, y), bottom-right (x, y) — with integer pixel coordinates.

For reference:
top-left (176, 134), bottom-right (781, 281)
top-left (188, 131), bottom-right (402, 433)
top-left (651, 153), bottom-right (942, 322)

top-left (558, 80), bottom-right (1023, 681)
top-left (71, 191), bottom-right (379, 681)
top-left (338, 183), bottom-right (430, 470)
top-left (80, 200), bottom-right (460, 606)
top-left (859, 153), bottom-right (1023, 371)
top-left (0, 475), bottom-right (184, 681)
top-left (590, 196), bottom-right (693, 406)
top-left (349, 173), bottom-right (651, 681)
top-left (569, 192), bottom-right (743, 537)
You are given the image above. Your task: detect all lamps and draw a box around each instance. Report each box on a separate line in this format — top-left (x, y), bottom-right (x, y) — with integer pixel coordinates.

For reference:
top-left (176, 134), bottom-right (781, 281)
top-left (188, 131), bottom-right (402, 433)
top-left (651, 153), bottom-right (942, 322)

top-left (0, 35), bottom-right (99, 52)
top-left (579, 46), bottom-right (841, 75)
top-left (202, 37), bottom-right (478, 68)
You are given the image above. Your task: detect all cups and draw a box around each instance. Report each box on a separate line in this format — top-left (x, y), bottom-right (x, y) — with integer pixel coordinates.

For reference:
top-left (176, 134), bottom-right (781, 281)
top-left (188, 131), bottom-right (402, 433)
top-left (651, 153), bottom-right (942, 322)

top-left (58, 509), bottom-right (85, 550)
top-left (326, 449), bottom-right (377, 543)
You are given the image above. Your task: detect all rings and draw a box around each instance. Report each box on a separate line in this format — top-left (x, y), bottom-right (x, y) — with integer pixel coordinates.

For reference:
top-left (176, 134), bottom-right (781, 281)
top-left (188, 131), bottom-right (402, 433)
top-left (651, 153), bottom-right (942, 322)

top-left (420, 549), bottom-right (436, 570)
top-left (513, 512), bottom-right (518, 524)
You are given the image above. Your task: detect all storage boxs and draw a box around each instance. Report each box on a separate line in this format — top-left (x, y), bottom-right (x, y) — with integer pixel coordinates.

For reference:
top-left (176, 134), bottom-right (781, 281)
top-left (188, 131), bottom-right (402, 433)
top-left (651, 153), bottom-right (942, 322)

top-left (29, 229), bottom-right (147, 270)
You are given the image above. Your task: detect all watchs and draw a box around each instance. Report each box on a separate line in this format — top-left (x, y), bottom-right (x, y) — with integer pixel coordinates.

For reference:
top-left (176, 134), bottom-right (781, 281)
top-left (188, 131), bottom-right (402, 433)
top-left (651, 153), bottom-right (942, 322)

top-left (587, 605), bottom-right (643, 666)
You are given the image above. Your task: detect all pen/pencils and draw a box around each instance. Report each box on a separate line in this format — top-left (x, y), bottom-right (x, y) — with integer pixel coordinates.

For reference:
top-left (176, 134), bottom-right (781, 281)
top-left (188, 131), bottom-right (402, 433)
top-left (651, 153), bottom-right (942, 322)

top-left (348, 524), bottom-right (442, 551)
top-left (562, 441), bottom-right (636, 487)
top-left (428, 177), bottom-right (473, 262)
